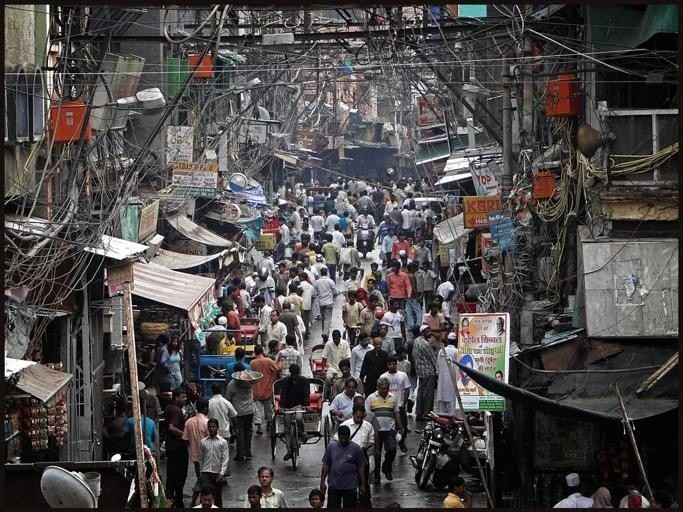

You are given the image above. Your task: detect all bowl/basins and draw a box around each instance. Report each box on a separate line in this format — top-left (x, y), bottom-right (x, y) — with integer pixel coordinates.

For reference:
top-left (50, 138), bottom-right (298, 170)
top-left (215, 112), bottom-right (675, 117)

top-left (230, 370), bottom-right (264, 385)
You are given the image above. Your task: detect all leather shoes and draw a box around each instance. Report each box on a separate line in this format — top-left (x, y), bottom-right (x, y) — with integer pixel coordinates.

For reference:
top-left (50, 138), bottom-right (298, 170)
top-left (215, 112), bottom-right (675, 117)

top-left (381, 467), bottom-right (394, 480)
top-left (398, 440), bottom-right (407, 452)
top-left (374, 472), bottom-right (380, 484)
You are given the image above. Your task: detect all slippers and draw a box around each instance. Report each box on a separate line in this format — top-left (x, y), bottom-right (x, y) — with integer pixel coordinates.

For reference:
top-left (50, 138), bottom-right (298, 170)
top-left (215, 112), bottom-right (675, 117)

top-left (255, 429), bottom-right (263, 434)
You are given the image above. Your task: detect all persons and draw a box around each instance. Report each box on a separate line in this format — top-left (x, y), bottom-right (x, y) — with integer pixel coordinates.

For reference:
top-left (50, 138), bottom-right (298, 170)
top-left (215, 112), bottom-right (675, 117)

top-left (495, 319), bottom-right (506, 338)
top-left (545, 472), bottom-right (674, 508)
top-left (494, 371), bottom-right (503, 384)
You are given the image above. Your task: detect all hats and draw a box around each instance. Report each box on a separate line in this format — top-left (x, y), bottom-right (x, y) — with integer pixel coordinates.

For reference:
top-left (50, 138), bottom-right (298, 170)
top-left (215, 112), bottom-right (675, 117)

top-left (566, 472), bottom-right (581, 487)
top-left (448, 332), bottom-right (456, 340)
top-left (419, 324), bottom-right (430, 333)
top-left (316, 254), bottom-right (323, 261)
top-left (218, 316), bottom-right (227, 325)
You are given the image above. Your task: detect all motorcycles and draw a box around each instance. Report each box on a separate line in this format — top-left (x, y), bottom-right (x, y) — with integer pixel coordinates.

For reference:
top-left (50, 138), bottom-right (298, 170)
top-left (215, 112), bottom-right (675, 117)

top-left (406, 411), bottom-right (465, 491)
top-left (355, 223), bottom-right (378, 258)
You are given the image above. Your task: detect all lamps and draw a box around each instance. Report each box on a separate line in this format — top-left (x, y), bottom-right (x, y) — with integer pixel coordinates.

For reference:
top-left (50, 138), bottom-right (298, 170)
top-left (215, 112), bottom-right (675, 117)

top-left (90, 87), bottom-right (166, 112)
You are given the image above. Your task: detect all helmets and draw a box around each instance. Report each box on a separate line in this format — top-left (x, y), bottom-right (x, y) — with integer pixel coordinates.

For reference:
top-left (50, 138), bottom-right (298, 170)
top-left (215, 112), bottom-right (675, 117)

top-left (258, 266), bottom-right (268, 281)
top-left (362, 205), bottom-right (369, 211)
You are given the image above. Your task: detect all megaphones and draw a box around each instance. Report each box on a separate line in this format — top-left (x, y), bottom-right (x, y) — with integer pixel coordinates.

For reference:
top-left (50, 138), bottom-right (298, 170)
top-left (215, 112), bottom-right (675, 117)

top-left (577, 122), bottom-right (616, 158)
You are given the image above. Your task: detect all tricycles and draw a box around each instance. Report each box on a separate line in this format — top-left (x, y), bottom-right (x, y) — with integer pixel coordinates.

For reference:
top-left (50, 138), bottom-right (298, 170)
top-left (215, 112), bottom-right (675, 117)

top-left (309, 344), bottom-right (338, 407)
top-left (268, 373), bottom-right (331, 461)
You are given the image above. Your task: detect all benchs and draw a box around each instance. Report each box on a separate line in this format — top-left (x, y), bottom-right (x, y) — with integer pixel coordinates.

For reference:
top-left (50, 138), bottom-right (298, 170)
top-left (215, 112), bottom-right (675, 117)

top-left (312, 358), bottom-right (322, 372)
top-left (274, 393), bottom-right (321, 413)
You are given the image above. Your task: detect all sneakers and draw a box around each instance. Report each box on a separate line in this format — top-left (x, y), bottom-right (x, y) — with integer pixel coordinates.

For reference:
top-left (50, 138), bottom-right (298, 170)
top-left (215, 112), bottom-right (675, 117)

top-left (232, 452), bottom-right (252, 461)
